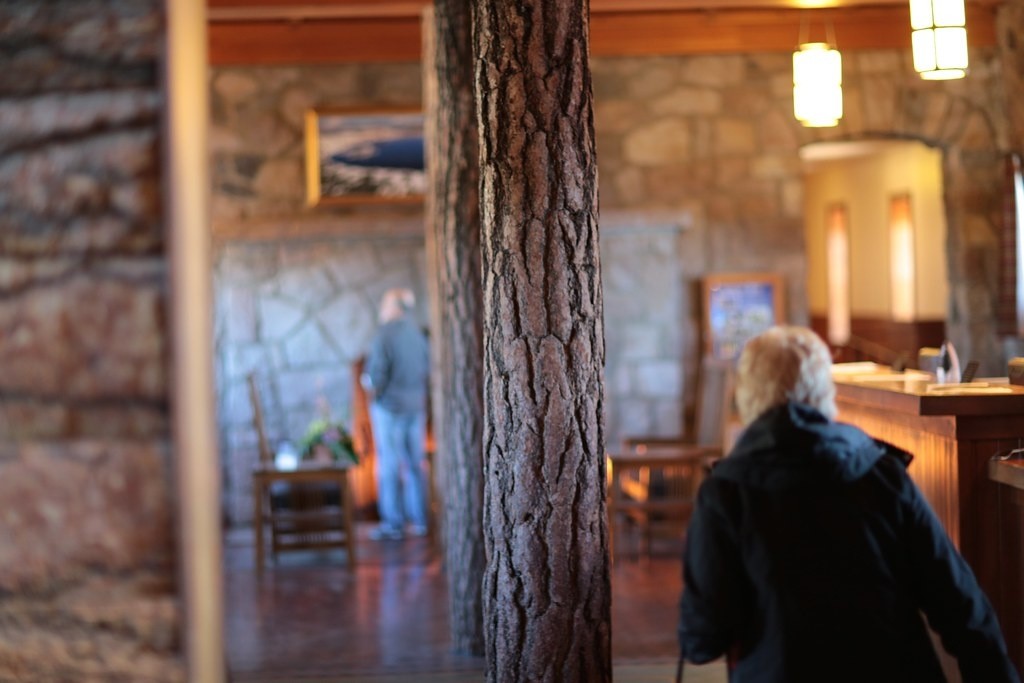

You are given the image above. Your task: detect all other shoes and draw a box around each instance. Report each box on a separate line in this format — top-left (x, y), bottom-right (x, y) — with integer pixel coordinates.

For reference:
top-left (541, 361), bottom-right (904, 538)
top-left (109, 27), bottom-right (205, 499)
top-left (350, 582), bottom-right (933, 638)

top-left (370, 526), bottom-right (402, 540)
top-left (407, 525), bottom-right (427, 536)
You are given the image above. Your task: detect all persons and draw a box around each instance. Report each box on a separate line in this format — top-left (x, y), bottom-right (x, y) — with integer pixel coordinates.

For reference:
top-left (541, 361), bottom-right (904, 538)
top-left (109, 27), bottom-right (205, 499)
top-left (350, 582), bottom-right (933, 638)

top-left (356, 289), bottom-right (429, 537)
top-left (676, 325), bottom-right (1021, 683)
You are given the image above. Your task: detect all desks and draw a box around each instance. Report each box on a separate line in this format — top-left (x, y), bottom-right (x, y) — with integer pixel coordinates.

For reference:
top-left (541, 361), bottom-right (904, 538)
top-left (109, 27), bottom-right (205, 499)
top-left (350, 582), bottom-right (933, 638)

top-left (249, 459), bottom-right (359, 567)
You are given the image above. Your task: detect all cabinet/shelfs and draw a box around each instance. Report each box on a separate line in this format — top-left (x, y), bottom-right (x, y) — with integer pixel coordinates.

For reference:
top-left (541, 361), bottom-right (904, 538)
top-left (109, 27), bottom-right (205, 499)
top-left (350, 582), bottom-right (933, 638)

top-left (806, 360), bottom-right (1023, 656)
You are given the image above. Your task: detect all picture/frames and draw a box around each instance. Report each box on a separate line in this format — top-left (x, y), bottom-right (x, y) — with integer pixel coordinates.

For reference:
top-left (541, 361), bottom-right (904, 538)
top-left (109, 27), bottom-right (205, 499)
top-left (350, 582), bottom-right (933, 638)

top-left (700, 272), bottom-right (789, 368)
top-left (304, 102), bottom-right (430, 208)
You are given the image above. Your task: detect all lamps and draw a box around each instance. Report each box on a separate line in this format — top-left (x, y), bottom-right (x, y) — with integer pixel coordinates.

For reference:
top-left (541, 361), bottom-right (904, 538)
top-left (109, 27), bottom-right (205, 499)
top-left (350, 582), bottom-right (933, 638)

top-left (783, 0), bottom-right (851, 127)
top-left (907, 0), bottom-right (969, 81)
